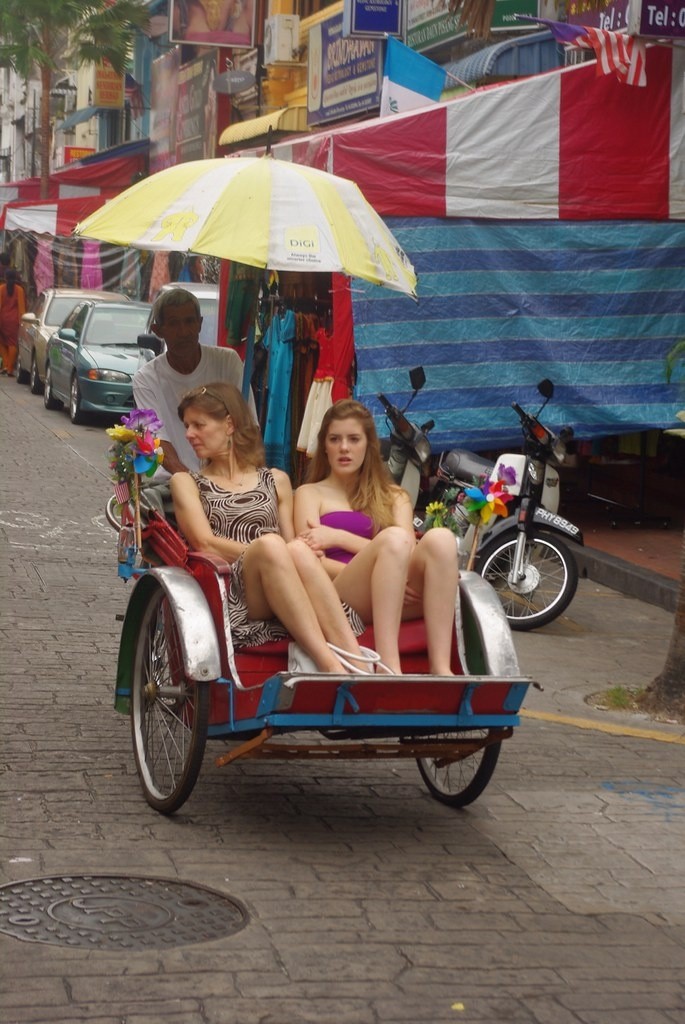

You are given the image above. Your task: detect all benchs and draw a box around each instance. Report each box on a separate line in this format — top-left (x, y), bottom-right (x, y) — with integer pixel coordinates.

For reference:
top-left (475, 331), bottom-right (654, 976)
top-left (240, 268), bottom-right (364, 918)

top-left (187, 551), bottom-right (462, 687)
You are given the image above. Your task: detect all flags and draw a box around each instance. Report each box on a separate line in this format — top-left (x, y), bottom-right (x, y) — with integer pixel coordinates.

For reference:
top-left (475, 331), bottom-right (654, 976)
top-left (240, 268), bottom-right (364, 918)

top-left (124, 72), bottom-right (145, 120)
top-left (380, 32), bottom-right (447, 117)
top-left (514, 16), bottom-right (651, 87)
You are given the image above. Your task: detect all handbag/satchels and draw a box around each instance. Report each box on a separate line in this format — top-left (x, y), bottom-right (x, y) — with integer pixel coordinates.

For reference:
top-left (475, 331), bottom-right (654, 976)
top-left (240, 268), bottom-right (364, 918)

top-left (287, 641), bottom-right (394, 675)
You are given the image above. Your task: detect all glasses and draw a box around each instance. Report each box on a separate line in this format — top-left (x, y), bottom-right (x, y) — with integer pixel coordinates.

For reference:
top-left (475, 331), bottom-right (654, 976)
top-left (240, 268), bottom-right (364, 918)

top-left (182, 388), bottom-right (223, 403)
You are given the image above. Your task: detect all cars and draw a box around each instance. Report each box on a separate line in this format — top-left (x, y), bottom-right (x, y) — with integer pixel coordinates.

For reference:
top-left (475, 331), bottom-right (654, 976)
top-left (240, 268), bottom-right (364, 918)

top-left (44, 300), bottom-right (155, 426)
top-left (16, 286), bottom-right (132, 396)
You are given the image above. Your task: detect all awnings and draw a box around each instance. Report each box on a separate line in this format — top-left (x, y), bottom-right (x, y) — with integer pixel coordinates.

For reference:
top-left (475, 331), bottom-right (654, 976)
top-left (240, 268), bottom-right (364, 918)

top-left (0, 74), bottom-right (151, 237)
top-left (219, 28), bottom-right (685, 221)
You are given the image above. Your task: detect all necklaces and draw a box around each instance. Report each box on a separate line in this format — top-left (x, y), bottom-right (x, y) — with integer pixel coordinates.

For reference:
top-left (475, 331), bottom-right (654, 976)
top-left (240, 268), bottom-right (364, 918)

top-left (202, 466), bottom-right (246, 487)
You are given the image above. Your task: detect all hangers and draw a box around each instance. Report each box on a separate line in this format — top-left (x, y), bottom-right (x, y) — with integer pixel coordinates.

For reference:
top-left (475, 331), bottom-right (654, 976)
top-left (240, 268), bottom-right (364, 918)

top-left (278, 296), bottom-right (331, 320)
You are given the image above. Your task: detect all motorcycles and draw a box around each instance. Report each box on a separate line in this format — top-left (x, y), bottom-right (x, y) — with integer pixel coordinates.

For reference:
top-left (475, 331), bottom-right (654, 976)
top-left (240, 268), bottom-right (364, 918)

top-left (425, 377), bottom-right (586, 632)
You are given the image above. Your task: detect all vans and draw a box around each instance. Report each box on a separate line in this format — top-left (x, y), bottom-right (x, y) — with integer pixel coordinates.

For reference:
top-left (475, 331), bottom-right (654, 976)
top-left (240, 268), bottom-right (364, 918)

top-left (136, 280), bottom-right (221, 371)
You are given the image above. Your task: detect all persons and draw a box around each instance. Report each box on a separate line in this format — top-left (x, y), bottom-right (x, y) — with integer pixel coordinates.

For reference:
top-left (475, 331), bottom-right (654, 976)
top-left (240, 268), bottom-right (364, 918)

top-left (131, 288), bottom-right (260, 477)
top-left (292, 400), bottom-right (459, 675)
top-left (171, 382), bottom-right (369, 675)
top-left (0, 252), bottom-right (26, 377)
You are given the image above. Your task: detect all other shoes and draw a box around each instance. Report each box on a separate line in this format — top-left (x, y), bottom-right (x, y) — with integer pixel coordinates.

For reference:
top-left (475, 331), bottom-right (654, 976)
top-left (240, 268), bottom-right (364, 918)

top-left (7, 371), bottom-right (16, 377)
top-left (0, 368), bottom-right (7, 373)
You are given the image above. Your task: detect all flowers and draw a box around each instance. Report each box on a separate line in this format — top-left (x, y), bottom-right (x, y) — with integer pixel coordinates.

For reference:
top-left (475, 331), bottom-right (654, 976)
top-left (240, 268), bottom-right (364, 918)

top-left (106, 409), bottom-right (164, 525)
top-left (425, 463), bottom-right (517, 530)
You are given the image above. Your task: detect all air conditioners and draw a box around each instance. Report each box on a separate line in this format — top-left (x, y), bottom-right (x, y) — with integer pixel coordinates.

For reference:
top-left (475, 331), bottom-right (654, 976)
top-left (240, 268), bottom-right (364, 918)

top-left (262, 13), bottom-right (299, 66)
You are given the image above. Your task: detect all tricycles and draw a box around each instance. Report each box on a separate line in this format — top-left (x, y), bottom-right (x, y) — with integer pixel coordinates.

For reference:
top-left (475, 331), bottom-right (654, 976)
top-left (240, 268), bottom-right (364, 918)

top-left (107, 479), bottom-right (543, 815)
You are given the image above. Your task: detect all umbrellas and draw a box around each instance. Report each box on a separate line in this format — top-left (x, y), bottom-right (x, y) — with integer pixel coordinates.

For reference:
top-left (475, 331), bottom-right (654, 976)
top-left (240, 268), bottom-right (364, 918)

top-left (72, 124), bottom-right (419, 402)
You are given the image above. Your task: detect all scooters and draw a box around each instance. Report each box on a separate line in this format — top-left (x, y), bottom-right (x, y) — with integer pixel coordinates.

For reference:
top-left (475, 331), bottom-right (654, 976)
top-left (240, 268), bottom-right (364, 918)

top-left (377, 364), bottom-right (436, 534)
